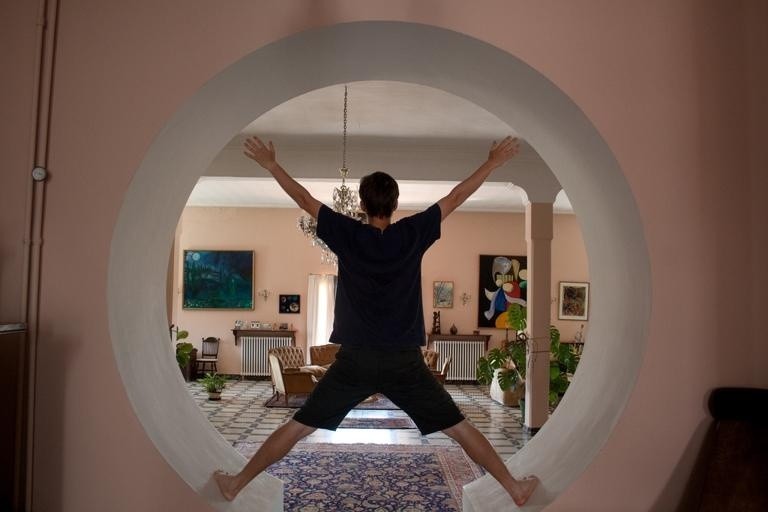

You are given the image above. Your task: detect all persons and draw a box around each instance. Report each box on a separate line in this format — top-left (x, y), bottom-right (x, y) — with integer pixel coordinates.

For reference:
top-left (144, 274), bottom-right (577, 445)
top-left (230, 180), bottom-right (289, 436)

top-left (213, 133), bottom-right (542, 507)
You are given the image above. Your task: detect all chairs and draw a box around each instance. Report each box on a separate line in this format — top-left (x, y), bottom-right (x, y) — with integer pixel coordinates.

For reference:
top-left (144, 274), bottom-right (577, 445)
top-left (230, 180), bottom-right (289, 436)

top-left (193, 336), bottom-right (221, 380)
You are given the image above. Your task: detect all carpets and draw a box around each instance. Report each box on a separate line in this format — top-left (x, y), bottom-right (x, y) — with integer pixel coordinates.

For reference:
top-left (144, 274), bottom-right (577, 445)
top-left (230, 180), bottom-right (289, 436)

top-left (337, 417), bottom-right (419, 429)
top-left (263, 392), bottom-right (402, 410)
top-left (232, 438), bottom-right (487, 512)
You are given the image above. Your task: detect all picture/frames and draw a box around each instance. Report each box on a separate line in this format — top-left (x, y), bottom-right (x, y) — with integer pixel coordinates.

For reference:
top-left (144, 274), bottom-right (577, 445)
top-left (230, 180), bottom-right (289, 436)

top-left (432, 281), bottom-right (454, 309)
top-left (181, 248), bottom-right (256, 311)
top-left (558, 281), bottom-right (590, 321)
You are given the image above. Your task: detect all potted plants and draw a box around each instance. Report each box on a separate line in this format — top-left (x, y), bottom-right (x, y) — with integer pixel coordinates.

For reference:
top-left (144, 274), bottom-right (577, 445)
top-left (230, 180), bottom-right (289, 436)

top-left (476, 302), bottom-right (581, 419)
top-left (196, 370), bottom-right (231, 401)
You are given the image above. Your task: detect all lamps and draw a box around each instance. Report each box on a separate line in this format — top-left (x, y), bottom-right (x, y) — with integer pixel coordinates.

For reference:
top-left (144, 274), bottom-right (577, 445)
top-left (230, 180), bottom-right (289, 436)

top-left (295, 84), bottom-right (368, 267)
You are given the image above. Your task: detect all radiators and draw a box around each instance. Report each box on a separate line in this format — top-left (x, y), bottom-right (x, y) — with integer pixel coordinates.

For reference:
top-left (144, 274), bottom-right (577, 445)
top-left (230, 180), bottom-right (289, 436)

top-left (240, 336), bottom-right (293, 380)
top-left (433, 340), bottom-right (485, 384)
